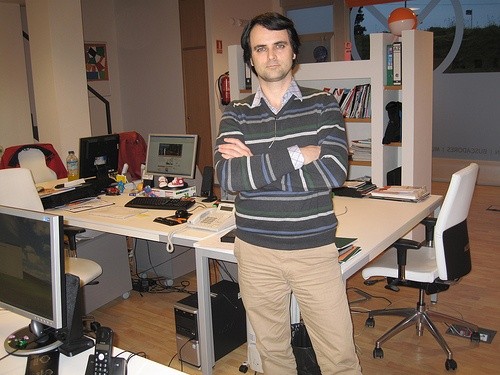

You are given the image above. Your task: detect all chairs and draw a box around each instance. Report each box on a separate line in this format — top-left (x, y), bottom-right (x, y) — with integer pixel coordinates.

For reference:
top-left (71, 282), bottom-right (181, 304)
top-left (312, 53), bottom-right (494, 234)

top-left (4, 143), bottom-right (87, 258)
top-left (0, 168), bottom-right (103, 334)
top-left (361, 162), bottom-right (479, 371)
top-left (116, 131), bottom-right (147, 183)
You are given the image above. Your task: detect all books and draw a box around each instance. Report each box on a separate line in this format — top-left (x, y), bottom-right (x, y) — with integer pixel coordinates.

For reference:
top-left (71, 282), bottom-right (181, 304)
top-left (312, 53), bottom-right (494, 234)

top-left (324, 84), bottom-right (371, 119)
top-left (334, 238), bottom-right (362, 264)
top-left (386, 42), bottom-right (402, 86)
top-left (351, 139), bottom-right (371, 161)
top-left (332, 181), bottom-right (429, 203)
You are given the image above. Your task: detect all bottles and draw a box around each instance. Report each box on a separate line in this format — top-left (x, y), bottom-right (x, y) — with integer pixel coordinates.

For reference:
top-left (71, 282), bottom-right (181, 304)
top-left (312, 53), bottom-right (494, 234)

top-left (65, 151), bottom-right (80, 181)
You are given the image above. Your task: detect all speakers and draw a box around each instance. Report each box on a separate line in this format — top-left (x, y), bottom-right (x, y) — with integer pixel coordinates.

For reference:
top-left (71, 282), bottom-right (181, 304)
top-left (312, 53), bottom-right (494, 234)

top-left (201, 166), bottom-right (217, 203)
top-left (56, 272), bottom-right (94, 357)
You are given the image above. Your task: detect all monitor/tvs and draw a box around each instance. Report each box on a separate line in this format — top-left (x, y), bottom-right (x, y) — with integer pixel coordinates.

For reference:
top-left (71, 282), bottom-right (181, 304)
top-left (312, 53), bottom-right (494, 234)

top-left (144, 134), bottom-right (199, 190)
top-left (79, 134), bottom-right (120, 187)
top-left (0, 204), bottom-right (68, 357)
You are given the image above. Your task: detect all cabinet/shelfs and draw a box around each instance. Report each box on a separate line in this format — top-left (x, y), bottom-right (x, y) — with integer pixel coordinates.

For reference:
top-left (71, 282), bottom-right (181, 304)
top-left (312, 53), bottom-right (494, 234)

top-left (228, 28), bottom-right (434, 244)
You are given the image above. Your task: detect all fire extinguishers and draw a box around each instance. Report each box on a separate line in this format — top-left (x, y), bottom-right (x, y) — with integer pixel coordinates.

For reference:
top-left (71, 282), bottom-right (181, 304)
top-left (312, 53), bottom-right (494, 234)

top-left (217, 71), bottom-right (231, 105)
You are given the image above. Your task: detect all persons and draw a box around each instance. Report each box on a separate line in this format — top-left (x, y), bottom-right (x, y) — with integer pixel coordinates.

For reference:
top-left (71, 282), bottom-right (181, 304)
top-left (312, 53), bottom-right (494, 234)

top-left (214, 12), bottom-right (362, 375)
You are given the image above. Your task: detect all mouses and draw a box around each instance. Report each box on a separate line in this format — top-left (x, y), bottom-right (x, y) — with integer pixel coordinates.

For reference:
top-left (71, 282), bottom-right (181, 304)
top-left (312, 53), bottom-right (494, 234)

top-left (175, 208), bottom-right (188, 218)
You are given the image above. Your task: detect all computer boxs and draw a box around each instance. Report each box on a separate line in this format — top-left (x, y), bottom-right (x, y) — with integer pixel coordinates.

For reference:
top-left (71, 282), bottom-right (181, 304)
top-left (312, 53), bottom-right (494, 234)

top-left (174, 279), bottom-right (247, 370)
top-left (63, 232), bottom-right (133, 315)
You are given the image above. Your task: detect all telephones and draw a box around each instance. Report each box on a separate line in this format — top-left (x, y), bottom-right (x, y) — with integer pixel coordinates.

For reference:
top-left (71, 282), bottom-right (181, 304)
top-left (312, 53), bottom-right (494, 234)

top-left (189, 203), bottom-right (236, 232)
top-left (84, 326), bottom-right (128, 375)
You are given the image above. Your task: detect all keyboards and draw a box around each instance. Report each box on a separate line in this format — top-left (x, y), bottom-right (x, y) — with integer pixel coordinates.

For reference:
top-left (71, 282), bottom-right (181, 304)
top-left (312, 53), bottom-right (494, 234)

top-left (124, 197), bottom-right (196, 210)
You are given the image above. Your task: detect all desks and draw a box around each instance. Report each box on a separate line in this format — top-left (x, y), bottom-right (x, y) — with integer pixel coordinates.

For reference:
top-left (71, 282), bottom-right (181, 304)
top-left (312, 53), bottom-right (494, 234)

top-left (0, 175), bottom-right (444, 375)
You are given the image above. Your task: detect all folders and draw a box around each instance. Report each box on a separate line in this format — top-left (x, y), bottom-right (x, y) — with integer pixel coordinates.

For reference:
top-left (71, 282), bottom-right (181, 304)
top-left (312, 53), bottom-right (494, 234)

top-left (386, 42), bottom-right (401, 86)
